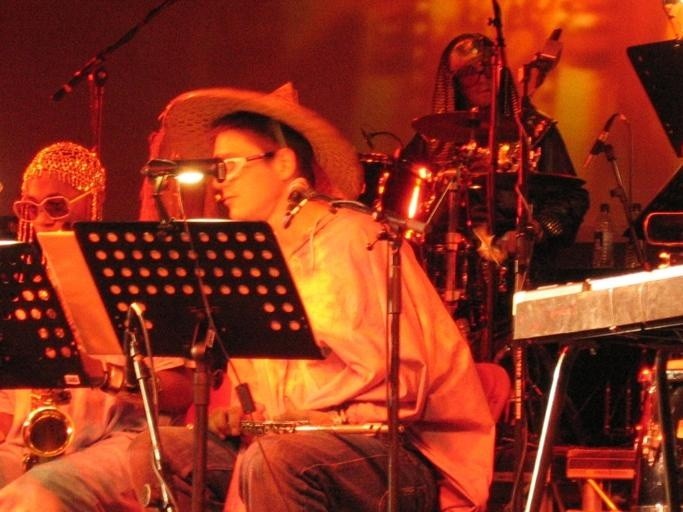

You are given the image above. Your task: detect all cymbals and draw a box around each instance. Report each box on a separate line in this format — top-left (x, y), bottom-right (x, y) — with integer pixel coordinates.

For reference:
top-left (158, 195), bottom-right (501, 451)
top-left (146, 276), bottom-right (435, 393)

top-left (410, 112), bottom-right (522, 145)
top-left (471, 174), bottom-right (586, 189)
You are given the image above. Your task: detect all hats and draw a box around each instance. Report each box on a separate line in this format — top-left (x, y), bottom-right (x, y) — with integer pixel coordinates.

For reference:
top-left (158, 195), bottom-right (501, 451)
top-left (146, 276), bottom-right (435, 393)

top-left (18, 141), bottom-right (106, 201)
top-left (161, 87), bottom-right (365, 207)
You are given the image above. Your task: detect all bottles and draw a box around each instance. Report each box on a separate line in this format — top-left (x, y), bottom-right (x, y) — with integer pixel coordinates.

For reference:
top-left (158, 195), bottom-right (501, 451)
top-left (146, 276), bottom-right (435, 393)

top-left (622, 202), bottom-right (649, 271)
top-left (590, 201), bottom-right (616, 271)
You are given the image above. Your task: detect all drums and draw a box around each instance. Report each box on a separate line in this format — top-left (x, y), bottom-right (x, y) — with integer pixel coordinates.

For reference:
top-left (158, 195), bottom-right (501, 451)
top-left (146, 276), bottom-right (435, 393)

top-left (424, 243), bottom-right (477, 301)
top-left (357, 154), bottom-right (436, 233)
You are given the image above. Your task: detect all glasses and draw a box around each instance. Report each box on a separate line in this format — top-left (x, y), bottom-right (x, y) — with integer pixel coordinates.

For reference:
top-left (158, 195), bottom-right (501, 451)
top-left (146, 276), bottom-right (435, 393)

top-left (11, 188), bottom-right (90, 224)
top-left (211, 151), bottom-right (272, 189)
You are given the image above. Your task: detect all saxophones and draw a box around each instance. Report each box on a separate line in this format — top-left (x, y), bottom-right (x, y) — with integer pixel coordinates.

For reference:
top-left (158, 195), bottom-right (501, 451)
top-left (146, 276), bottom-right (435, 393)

top-left (21, 390), bottom-right (74, 472)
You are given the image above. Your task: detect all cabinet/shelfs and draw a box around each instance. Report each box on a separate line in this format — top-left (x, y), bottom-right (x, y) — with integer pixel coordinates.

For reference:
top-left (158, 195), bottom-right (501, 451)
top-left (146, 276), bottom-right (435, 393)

top-left (525, 315), bottom-right (683, 512)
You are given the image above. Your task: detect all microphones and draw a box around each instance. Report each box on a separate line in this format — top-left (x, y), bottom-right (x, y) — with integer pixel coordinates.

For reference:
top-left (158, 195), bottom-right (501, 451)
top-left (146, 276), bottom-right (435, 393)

top-left (139, 154), bottom-right (229, 186)
top-left (583, 105), bottom-right (623, 174)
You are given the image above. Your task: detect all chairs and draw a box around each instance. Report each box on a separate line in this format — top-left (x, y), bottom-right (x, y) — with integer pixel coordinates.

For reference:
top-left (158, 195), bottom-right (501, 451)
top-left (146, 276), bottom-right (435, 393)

top-left (477, 360), bottom-right (527, 511)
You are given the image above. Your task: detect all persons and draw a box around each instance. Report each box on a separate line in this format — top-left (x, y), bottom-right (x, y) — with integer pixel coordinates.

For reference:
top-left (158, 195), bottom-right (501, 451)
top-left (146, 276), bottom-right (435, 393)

top-left (379, 30), bottom-right (593, 448)
top-left (126, 80), bottom-right (499, 512)
top-left (0, 141), bottom-right (192, 512)
